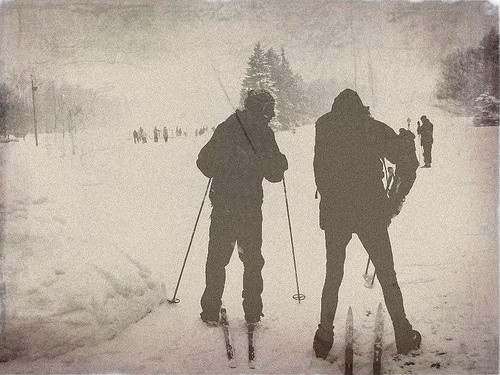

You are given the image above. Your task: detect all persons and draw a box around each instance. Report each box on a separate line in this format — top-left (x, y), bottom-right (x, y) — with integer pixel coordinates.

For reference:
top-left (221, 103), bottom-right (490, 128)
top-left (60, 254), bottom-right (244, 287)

top-left (132, 126), bottom-right (169, 144)
top-left (310, 88), bottom-right (421, 358)
top-left (195, 88), bottom-right (288, 324)
top-left (417, 113), bottom-right (434, 169)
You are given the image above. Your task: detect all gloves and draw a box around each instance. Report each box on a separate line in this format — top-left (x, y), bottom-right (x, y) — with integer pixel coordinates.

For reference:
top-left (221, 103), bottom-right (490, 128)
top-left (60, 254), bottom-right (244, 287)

top-left (389, 196), bottom-right (406, 218)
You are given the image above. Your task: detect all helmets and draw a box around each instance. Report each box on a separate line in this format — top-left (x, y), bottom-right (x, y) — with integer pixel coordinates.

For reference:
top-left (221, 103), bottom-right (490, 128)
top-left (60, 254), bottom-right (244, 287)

top-left (245, 88), bottom-right (277, 123)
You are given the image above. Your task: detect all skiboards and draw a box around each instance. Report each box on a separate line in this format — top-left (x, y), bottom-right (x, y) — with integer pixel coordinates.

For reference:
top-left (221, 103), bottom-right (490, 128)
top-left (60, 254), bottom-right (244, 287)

top-left (344, 302), bottom-right (384, 375)
top-left (221, 308), bottom-right (256, 369)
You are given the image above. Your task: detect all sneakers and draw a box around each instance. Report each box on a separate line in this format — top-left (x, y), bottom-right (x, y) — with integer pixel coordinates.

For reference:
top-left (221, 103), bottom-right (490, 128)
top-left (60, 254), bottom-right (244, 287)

top-left (200, 308), bottom-right (219, 326)
top-left (398, 330), bottom-right (422, 353)
top-left (245, 305), bottom-right (263, 323)
top-left (313, 330), bottom-right (334, 359)
